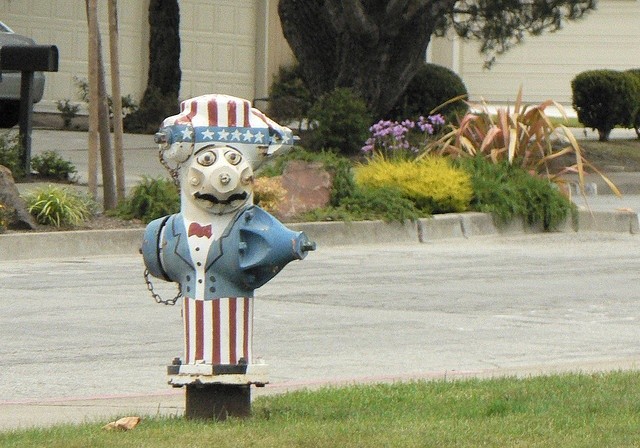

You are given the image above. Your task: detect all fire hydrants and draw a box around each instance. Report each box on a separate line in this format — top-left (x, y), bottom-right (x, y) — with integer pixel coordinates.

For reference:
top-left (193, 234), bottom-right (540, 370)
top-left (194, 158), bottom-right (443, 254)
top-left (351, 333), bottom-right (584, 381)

top-left (137, 93), bottom-right (317, 419)
